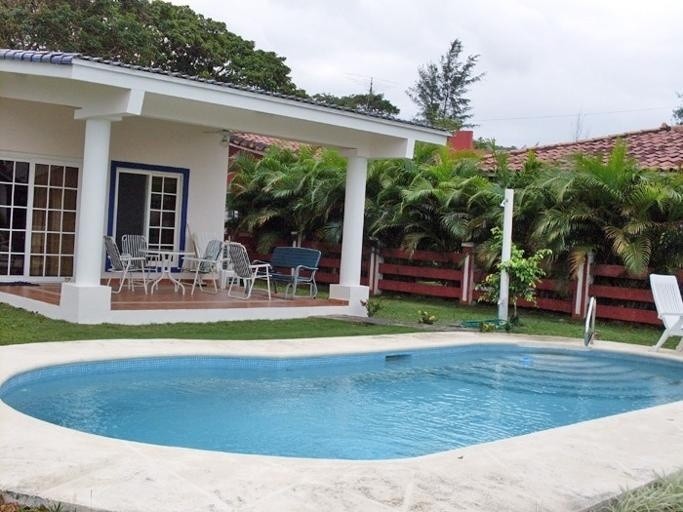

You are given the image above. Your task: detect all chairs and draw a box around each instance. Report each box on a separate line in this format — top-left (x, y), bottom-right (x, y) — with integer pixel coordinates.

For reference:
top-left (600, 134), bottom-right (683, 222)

top-left (251, 247), bottom-right (321, 299)
top-left (649, 273), bottom-right (683, 352)
top-left (122, 234), bottom-right (159, 292)
top-left (105, 235), bottom-right (148, 295)
top-left (175, 239), bottom-right (223, 296)
top-left (227, 242), bottom-right (272, 301)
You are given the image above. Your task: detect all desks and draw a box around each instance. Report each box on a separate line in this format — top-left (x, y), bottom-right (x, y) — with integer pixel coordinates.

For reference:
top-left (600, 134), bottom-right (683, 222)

top-left (138, 248), bottom-right (195, 296)
